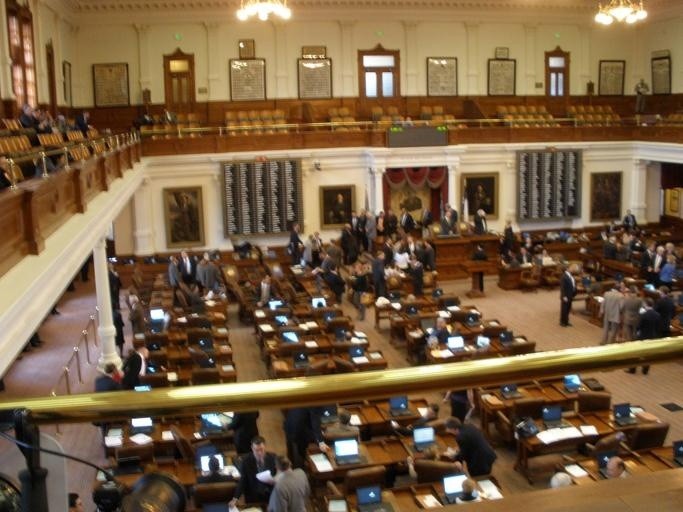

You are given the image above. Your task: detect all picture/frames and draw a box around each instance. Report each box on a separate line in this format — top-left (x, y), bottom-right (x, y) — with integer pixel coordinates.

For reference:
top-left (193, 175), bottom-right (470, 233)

top-left (588, 171), bottom-right (623, 223)
top-left (317, 184), bottom-right (357, 231)
top-left (62, 35), bottom-right (334, 107)
top-left (460, 171), bottom-right (499, 224)
top-left (423, 42), bottom-right (673, 97)
top-left (161, 185), bottom-right (207, 249)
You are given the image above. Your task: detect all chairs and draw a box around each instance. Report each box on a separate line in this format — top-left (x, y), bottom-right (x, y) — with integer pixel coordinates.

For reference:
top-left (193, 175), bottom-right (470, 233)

top-left (0, 110), bottom-right (140, 184)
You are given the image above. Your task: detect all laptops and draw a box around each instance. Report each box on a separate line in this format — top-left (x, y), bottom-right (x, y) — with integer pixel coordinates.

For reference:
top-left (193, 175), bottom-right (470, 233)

top-left (420, 318), bottom-right (438, 337)
top-left (390, 292), bottom-right (402, 304)
top-left (542, 404), bottom-right (570, 430)
top-left (198, 453), bottom-right (225, 477)
top-left (432, 289), bottom-right (443, 298)
top-left (334, 327), bottom-right (345, 338)
top-left (348, 346), bottom-right (369, 363)
top-left (499, 329), bottom-right (517, 347)
top-left (476, 335), bottom-right (491, 348)
top-left (443, 474), bottom-right (471, 503)
top-left (613, 402), bottom-right (638, 427)
top-left (150, 308), bottom-right (163, 323)
top-left (313, 298), bottom-right (327, 310)
top-left (292, 351), bottom-right (311, 368)
top-left (320, 403), bottom-right (341, 426)
top-left (147, 358), bottom-right (165, 373)
top-left (598, 449), bottom-right (619, 479)
top-left (499, 383), bottom-right (524, 399)
top-left (199, 334), bottom-right (212, 351)
top-left (197, 355), bottom-right (215, 369)
top-left (465, 313), bottom-right (480, 327)
top-left (133, 385), bottom-right (151, 392)
top-left (129, 418), bottom-right (154, 436)
top-left (406, 305), bottom-right (418, 317)
top-left (275, 314), bottom-right (288, 325)
top-left (447, 336), bottom-right (470, 353)
top-left (412, 426), bottom-right (438, 452)
top-left (389, 395), bottom-right (414, 417)
top-left (334, 438), bottom-right (362, 464)
top-left (563, 374), bottom-right (585, 395)
top-left (145, 334), bottom-right (163, 350)
top-left (357, 483), bottom-right (389, 512)
top-left (268, 300), bottom-right (283, 310)
top-left (444, 301), bottom-right (455, 308)
top-left (672, 440), bottom-right (683, 466)
top-left (280, 331), bottom-right (299, 346)
top-left (324, 311), bottom-right (336, 323)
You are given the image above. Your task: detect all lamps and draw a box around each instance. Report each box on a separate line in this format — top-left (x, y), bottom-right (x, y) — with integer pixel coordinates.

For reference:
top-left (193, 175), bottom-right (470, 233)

top-left (233, 0), bottom-right (294, 23)
top-left (593, 1), bottom-right (647, 27)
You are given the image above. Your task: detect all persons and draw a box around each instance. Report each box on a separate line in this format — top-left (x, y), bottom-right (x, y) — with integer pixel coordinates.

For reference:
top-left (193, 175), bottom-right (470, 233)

top-left (634, 78), bottom-right (649, 112)
top-left (0, 100), bottom-right (177, 193)
top-left (170, 191), bottom-right (200, 242)
top-left (329, 192), bottom-right (348, 224)
top-left (471, 182), bottom-right (492, 214)
top-left (1, 205), bottom-right (683, 512)
